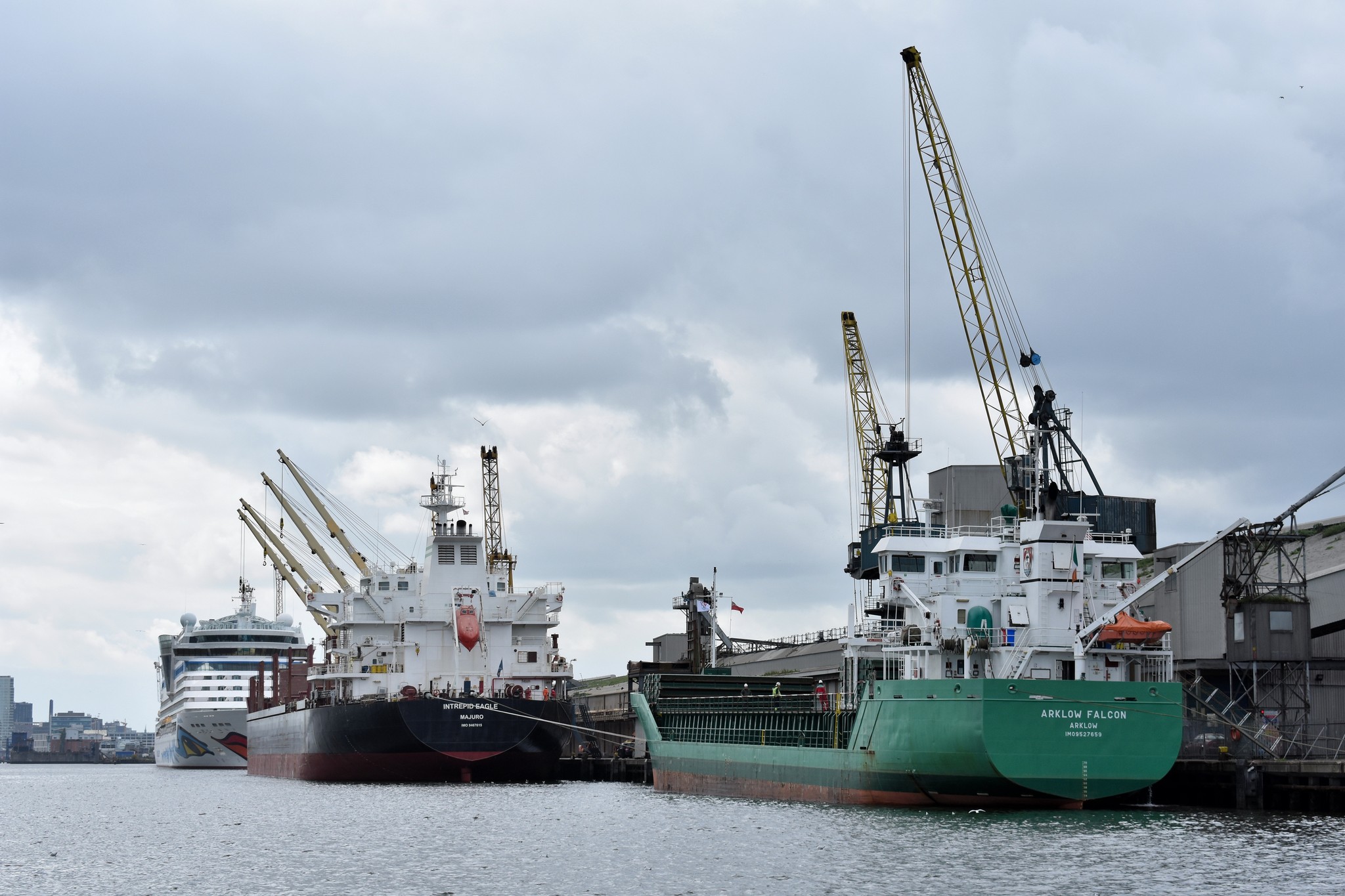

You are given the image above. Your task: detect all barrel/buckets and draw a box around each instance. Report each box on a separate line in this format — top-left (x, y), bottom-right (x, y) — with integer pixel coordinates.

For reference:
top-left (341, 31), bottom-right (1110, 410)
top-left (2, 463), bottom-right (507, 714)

top-left (377, 665), bottom-right (382, 673)
top-left (371, 665), bottom-right (377, 673)
top-left (362, 666), bottom-right (365, 673)
top-left (382, 664), bottom-right (387, 673)
top-left (364, 666), bottom-right (370, 673)
top-left (389, 664), bottom-right (403, 673)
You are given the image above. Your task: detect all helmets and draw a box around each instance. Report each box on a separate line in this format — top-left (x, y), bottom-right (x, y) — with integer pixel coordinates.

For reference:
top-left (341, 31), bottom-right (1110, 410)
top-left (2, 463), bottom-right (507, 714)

top-left (776, 682), bottom-right (781, 686)
top-left (819, 680), bottom-right (822, 683)
top-left (744, 684), bottom-right (748, 686)
top-left (528, 686), bottom-right (530, 687)
top-left (552, 687), bottom-right (553, 689)
top-left (545, 686), bottom-right (547, 688)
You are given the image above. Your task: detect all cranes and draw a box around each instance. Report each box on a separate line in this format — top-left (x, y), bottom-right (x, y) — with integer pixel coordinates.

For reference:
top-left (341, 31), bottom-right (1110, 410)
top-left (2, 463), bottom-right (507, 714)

top-left (898, 46), bottom-right (1159, 557)
top-left (837, 308), bottom-right (936, 579)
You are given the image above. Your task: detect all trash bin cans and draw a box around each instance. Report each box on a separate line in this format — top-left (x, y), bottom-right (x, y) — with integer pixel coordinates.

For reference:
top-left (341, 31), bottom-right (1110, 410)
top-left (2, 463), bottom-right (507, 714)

top-left (364, 666), bottom-right (370, 673)
top-left (397, 664), bottom-right (402, 673)
top-left (382, 665), bottom-right (387, 673)
top-left (377, 665), bottom-right (382, 673)
top-left (371, 665), bottom-right (377, 673)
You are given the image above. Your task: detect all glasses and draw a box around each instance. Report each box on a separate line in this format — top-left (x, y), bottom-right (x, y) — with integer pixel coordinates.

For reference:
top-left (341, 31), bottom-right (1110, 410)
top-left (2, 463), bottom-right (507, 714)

top-left (745, 686), bottom-right (747, 687)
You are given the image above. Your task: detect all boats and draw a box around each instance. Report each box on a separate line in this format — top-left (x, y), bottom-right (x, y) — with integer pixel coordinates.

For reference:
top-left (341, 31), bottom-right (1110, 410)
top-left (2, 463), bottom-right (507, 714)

top-left (630, 563), bottom-right (1186, 809)
top-left (152, 576), bottom-right (311, 773)
top-left (234, 446), bottom-right (580, 785)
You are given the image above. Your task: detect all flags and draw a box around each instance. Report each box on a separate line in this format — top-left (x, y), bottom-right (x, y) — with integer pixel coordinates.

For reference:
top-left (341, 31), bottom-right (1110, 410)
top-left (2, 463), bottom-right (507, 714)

top-left (1070, 540), bottom-right (1078, 583)
